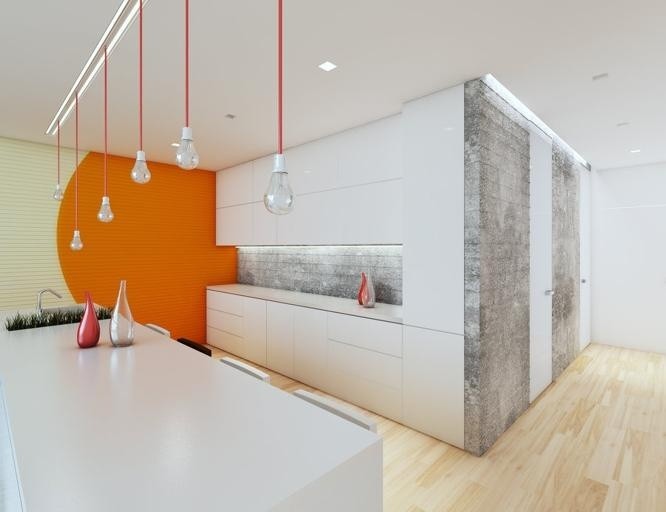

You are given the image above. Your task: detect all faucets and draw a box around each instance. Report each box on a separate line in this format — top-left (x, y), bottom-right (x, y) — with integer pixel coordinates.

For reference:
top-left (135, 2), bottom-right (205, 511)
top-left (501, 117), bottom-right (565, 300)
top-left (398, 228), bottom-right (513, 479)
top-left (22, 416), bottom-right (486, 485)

top-left (35, 288), bottom-right (64, 312)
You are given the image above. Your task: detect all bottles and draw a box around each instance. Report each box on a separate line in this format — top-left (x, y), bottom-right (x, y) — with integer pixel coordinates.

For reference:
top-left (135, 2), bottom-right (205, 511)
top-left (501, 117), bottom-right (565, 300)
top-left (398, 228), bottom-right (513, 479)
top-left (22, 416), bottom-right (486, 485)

top-left (76, 279), bottom-right (133, 348)
top-left (357, 272), bottom-right (375, 308)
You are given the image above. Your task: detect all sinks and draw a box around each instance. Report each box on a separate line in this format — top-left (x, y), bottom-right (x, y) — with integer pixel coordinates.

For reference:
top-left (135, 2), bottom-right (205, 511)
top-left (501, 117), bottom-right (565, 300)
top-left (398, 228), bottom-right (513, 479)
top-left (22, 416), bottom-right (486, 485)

top-left (40, 304), bottom-right (84, 314)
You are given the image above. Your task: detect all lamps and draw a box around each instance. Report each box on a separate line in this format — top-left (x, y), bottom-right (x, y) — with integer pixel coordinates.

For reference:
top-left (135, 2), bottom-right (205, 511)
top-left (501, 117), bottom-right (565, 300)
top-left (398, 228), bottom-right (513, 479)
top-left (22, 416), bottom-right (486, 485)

top-left (41, 0), bottom-right (296, 251)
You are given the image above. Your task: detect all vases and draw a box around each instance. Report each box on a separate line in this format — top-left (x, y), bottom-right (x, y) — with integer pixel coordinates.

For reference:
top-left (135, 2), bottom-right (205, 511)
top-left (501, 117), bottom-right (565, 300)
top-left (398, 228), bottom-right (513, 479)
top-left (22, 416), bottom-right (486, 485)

top-left (76, 280), bottom-right (134, 347)
